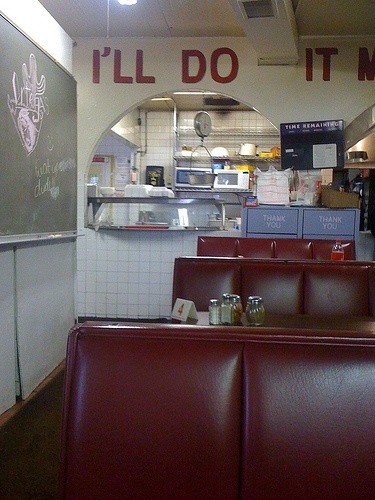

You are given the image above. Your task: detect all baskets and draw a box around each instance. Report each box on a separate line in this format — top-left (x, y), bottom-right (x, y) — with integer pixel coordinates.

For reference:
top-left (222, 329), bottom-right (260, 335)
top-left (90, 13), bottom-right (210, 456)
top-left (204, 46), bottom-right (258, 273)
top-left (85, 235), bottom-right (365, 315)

top-left (321, 189), bottom-right (359, 208)
top-left (321, 182), bottom-right (333, 191)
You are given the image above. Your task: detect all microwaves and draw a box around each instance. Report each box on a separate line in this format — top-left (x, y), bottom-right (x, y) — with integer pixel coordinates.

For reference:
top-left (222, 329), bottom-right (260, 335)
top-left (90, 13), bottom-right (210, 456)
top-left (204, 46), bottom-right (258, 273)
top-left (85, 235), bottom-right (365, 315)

top-left (212, 169), bottom-right (250, 189)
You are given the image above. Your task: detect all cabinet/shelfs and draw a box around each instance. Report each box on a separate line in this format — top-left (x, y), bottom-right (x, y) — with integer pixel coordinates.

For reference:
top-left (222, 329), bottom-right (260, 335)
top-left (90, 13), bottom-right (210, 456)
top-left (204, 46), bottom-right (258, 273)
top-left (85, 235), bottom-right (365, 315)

top-left (239, 205), bottom-right (361, 262)
top-left (171, 91), bottom-right (281, 190)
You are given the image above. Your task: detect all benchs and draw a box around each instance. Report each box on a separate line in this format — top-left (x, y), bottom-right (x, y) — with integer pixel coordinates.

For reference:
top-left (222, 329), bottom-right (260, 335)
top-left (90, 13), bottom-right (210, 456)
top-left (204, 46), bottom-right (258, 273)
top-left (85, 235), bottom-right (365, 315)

top-left (50, 235), bottom-right (375, 500)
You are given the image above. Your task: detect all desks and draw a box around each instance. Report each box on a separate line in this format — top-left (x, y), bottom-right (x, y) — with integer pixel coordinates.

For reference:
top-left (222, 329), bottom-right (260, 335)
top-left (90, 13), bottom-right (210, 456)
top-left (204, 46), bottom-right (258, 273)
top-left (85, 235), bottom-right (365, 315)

top-left (174, 310), bottom-right (375, 334)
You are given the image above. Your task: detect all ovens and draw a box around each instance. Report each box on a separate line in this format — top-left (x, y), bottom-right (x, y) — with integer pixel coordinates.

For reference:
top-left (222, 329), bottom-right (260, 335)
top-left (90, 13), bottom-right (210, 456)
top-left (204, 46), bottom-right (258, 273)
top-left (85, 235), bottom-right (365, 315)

top-left (175, 167), bottom-right (212, 189)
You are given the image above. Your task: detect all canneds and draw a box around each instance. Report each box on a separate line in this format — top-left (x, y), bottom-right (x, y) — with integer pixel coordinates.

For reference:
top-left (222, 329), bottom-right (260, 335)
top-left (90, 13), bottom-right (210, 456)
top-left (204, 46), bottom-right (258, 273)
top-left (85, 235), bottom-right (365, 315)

top-left (230, 295), bottom-right (242, 322)
top-left (246, 296), bottom-right (264, 326)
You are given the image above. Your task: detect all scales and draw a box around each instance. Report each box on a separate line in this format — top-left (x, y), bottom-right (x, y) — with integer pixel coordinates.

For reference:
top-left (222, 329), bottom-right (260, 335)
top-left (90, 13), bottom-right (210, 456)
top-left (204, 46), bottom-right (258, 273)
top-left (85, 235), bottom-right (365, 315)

top-left (185, 112), bottom-right (218, 186)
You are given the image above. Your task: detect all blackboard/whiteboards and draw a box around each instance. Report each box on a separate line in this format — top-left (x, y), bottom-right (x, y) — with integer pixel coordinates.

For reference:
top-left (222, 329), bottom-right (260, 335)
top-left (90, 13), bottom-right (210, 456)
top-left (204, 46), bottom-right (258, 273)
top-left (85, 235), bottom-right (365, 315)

top-left (0, 10), bottom-right (77, 244)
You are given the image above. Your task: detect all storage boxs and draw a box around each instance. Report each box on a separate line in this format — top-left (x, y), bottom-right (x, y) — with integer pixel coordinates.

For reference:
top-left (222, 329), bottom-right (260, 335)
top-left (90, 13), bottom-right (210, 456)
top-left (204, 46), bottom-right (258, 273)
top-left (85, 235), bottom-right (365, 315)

top-left (321, 190), bottom-right (360, 208)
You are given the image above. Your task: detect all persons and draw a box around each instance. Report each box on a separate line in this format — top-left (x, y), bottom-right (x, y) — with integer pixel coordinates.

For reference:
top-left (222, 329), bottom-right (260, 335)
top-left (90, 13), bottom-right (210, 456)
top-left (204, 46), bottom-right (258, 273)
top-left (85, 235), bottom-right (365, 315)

top-left (339, 181), bottom-right (347, 192)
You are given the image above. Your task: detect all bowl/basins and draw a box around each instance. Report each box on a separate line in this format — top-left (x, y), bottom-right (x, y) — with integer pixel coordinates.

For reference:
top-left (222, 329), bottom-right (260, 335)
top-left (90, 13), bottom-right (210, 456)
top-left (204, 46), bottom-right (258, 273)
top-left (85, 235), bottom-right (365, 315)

top-left (99, 186), bottom-right (115, 197)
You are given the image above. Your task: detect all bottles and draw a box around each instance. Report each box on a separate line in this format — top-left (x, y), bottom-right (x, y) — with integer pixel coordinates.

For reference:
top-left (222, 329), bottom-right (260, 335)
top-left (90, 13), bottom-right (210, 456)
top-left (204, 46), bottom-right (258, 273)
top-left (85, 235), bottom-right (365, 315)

top-left (219, 292), bottom-right (231, 324)
top-left (209, 300), bottom-right (220, 325)
top-left (245, 295), bottom-right (265, 326)
top-left (229, 294), bottom-right (244, 325)
top-left (331, 239), bottom-right (344, 261)
